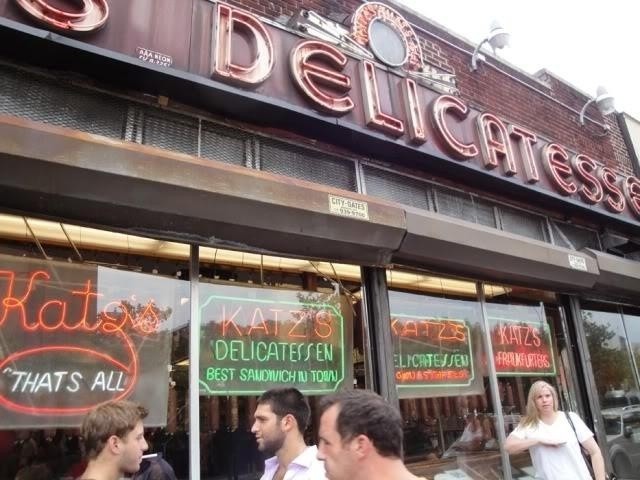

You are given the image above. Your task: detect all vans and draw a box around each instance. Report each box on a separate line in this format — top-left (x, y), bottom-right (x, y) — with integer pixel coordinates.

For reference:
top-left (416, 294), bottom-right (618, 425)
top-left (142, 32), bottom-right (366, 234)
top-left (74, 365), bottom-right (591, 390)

top-left (600, 390), bottom-right (640, 408)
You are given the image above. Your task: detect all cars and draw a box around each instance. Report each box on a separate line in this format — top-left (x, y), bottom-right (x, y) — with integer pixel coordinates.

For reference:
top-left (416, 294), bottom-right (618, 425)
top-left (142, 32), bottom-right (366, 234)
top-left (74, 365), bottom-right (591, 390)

top-left (602, 405), bottom-right (640, 475)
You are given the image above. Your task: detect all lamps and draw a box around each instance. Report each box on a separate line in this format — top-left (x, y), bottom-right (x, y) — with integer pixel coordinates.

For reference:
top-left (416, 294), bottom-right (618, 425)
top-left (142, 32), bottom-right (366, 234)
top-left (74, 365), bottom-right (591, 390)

top-left (471, 21), bottom-right (509, 73)
top-left (580, 85), bottom-right (617, 125)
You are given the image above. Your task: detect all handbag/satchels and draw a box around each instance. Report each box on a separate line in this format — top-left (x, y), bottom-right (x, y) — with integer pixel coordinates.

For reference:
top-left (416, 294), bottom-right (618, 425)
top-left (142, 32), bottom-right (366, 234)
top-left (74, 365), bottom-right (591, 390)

top-left (567, 411), bottom-right (617, 480)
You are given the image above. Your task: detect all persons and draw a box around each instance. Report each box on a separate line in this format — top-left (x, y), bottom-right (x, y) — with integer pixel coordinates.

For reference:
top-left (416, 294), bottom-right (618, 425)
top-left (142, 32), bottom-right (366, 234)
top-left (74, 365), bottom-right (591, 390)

top-left (505, 379), bottom-right (606, 480)
top-left (315, 390), bottom-right (427, 480)
top-left (250, 387), bottom-right (328, 479)
top-left (77, 401), bottom-right (149, 480)
top-left (0, 422), bottom-right (264, 480)
top-left (432, 383), bottom-right (504, 459)
